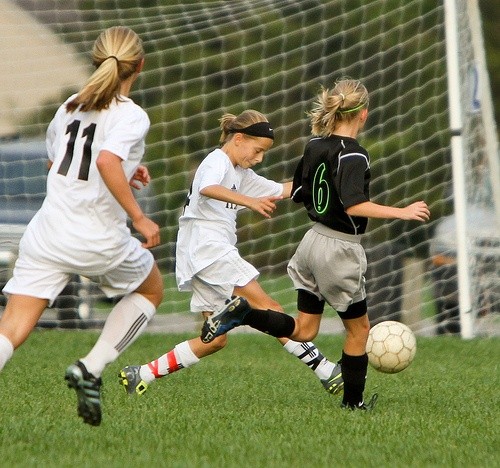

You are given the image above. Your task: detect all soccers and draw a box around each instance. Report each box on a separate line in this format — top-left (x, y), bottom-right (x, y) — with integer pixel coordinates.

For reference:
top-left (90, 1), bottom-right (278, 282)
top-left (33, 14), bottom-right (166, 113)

top-left (365, 321), bottom-right (416, 374)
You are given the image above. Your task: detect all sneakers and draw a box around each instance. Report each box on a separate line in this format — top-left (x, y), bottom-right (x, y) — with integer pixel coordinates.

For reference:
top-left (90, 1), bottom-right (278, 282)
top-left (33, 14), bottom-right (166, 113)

top-left (320, 364), bottom-right (343, 395)
top-left (119, 365), bottom-right (148, 397)
top-left (200, 295), bottom-right (250, 344)
top-left (64, 360), bottom-right (103, 426)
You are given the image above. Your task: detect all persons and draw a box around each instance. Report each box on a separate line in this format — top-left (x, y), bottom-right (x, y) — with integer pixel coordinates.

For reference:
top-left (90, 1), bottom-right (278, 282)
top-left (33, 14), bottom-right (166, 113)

top-left (1, 25), bottom-right (166, 426)
top-left (201, 79), bottom-right (431, 412)
top-left (118, 109), bottom-right (345, 398)
